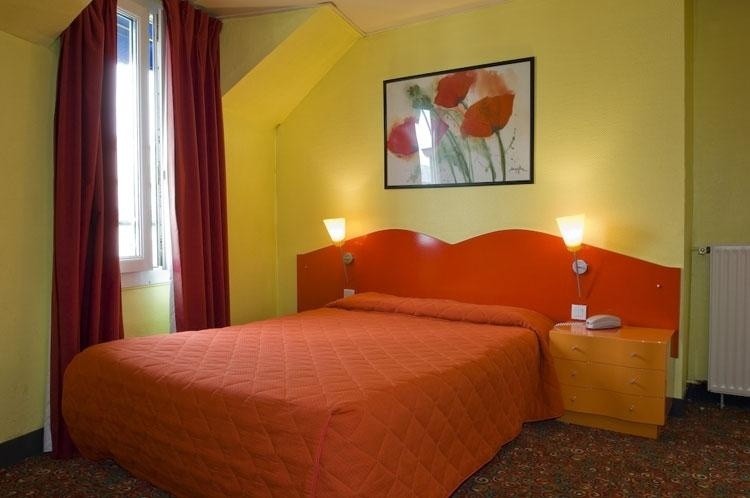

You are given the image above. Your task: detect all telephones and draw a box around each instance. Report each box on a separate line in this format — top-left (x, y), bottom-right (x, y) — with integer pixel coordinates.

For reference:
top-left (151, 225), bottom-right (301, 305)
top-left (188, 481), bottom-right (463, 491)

top-left (585, 314), bottom-right (621, 329)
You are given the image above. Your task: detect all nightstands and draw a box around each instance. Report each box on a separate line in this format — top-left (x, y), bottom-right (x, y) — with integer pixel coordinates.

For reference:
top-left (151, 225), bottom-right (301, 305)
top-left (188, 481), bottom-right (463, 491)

top-left (548, 320), bottom-right (675, 441)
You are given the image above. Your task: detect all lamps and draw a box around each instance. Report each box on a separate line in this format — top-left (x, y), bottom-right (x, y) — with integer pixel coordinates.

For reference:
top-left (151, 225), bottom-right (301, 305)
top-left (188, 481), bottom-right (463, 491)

top-left (557, 215), bottom-right (593, 296)
top-left (323, 216), bottom-right (355, 283)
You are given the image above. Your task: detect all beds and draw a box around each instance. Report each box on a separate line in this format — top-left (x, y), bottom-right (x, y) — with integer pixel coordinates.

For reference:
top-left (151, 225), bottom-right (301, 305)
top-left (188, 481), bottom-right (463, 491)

top-left (60, 292), bottom-right (556, 498)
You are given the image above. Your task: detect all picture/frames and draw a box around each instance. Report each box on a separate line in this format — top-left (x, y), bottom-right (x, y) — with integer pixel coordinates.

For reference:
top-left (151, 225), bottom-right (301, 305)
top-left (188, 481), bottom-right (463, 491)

top-left (383, 56), bottom-right (534, 189)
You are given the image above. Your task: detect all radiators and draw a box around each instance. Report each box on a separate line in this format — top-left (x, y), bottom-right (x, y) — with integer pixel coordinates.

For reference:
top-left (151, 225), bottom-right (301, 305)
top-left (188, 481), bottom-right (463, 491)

top-left (698, 242), bottom-right (749, 412)
